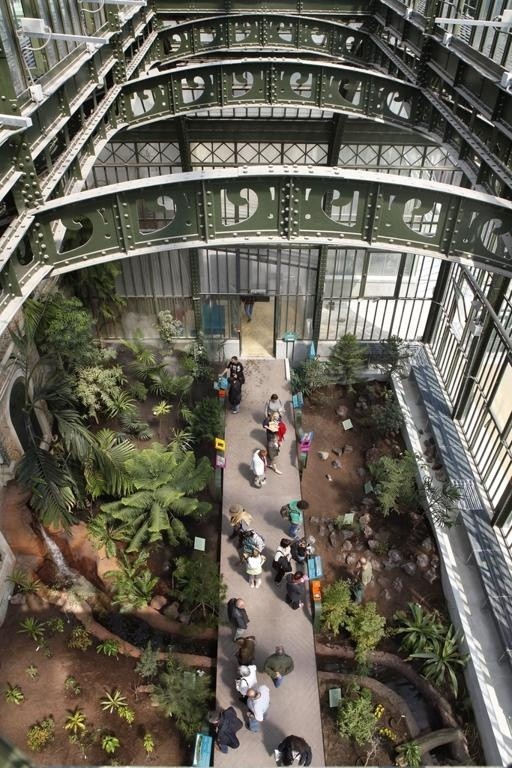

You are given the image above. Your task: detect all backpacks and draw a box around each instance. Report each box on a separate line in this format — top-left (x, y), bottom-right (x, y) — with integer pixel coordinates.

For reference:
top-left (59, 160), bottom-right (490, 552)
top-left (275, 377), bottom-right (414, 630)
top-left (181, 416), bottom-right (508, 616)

top-left (280, 504), bottom-right (291, 523)
top-left (272, 559), bottom-right (280, 570)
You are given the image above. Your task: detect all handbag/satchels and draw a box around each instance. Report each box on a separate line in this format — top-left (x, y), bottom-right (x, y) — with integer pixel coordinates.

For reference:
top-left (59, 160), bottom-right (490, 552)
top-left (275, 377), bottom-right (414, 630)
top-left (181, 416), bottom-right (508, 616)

top-left (253, 479), bottom-right (262, 489)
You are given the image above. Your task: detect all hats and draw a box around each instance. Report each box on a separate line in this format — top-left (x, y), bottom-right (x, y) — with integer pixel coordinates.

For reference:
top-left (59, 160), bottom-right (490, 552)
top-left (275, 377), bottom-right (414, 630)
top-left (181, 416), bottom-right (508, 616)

top-left (228, 503), bottom-right (244, 518)
